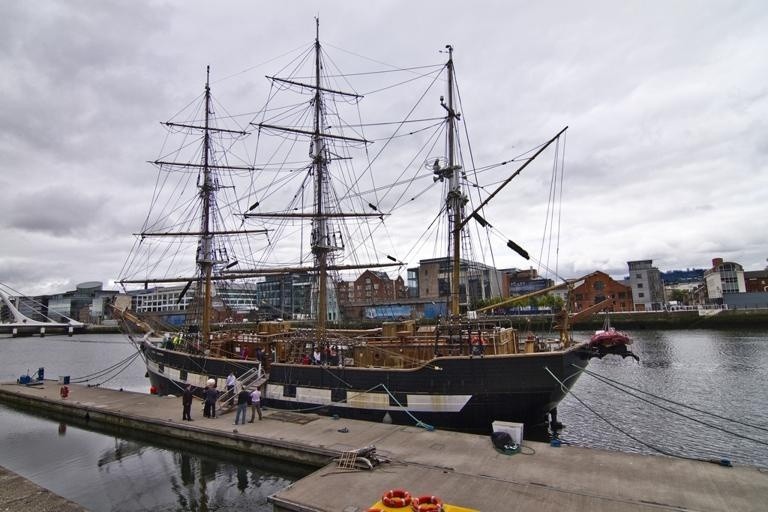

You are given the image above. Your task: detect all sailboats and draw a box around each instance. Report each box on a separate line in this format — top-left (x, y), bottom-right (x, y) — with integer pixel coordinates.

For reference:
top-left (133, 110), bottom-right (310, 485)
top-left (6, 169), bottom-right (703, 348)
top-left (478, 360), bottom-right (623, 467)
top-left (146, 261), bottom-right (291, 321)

top-left (107, 15), bottom-right (639, 423)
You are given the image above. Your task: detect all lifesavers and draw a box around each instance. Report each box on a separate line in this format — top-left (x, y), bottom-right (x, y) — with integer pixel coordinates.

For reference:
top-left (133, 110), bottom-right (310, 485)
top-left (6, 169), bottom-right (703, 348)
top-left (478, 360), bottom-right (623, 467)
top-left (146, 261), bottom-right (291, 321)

top-left (472, 337), bottom-right (484, 344)
top-left (372, 351), bottom-right (383, 361)
top-left (412, 496), bottom-right (443, 512)
top-left (363, 509), bottom-right (385, 512)
top-left (382, 489), bottom-right (410, 507)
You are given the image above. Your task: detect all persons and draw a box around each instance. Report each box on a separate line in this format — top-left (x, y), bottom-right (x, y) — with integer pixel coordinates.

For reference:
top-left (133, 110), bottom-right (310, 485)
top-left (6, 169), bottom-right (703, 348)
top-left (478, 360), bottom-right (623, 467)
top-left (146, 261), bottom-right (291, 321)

top-left (231, 385), bottom-right (250, 426)
top-left (203, 384), bottom-right (218, 419)
top-left (166, 336), bottom-right (174, 350)
top-left (222, 462), bottom-right (235, 483)
top-left (249, 471), bottom-right (263, 488)
top-left (200, 386), bottom-right (210, 418)
top-left (301, 343), bottom-right (341, 367)
top-left (179, 454), bottom-right (195, 486)
top-left (253, 345), bottom-right (261, 363)
top-left (526, 330), bottom-right (534, 341)
top-left (247, 386), bottom-right (262, 424)
top-left (270, 346), bottom-right (275, 363)
top-left (235, 464), bottom-right (249, 499)
top-left (224, 369), bottom-right (237, 406)
top-left (259, 346), bottom-right (266, 378)
top-left (240, 343), bottom-right (250, 361)
top-left (172, 333), bottom-right (179, 349)
top-left (201, 456), bottom-right (217, 480)
top-left (181, 384), bottom-right (194, 421)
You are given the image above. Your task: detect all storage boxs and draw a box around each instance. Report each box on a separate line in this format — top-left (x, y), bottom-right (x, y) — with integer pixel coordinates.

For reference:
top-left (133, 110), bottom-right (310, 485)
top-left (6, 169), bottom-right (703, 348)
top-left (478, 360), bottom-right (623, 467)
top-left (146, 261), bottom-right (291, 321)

top-left (493, 421), bottom-right (524, 445)
